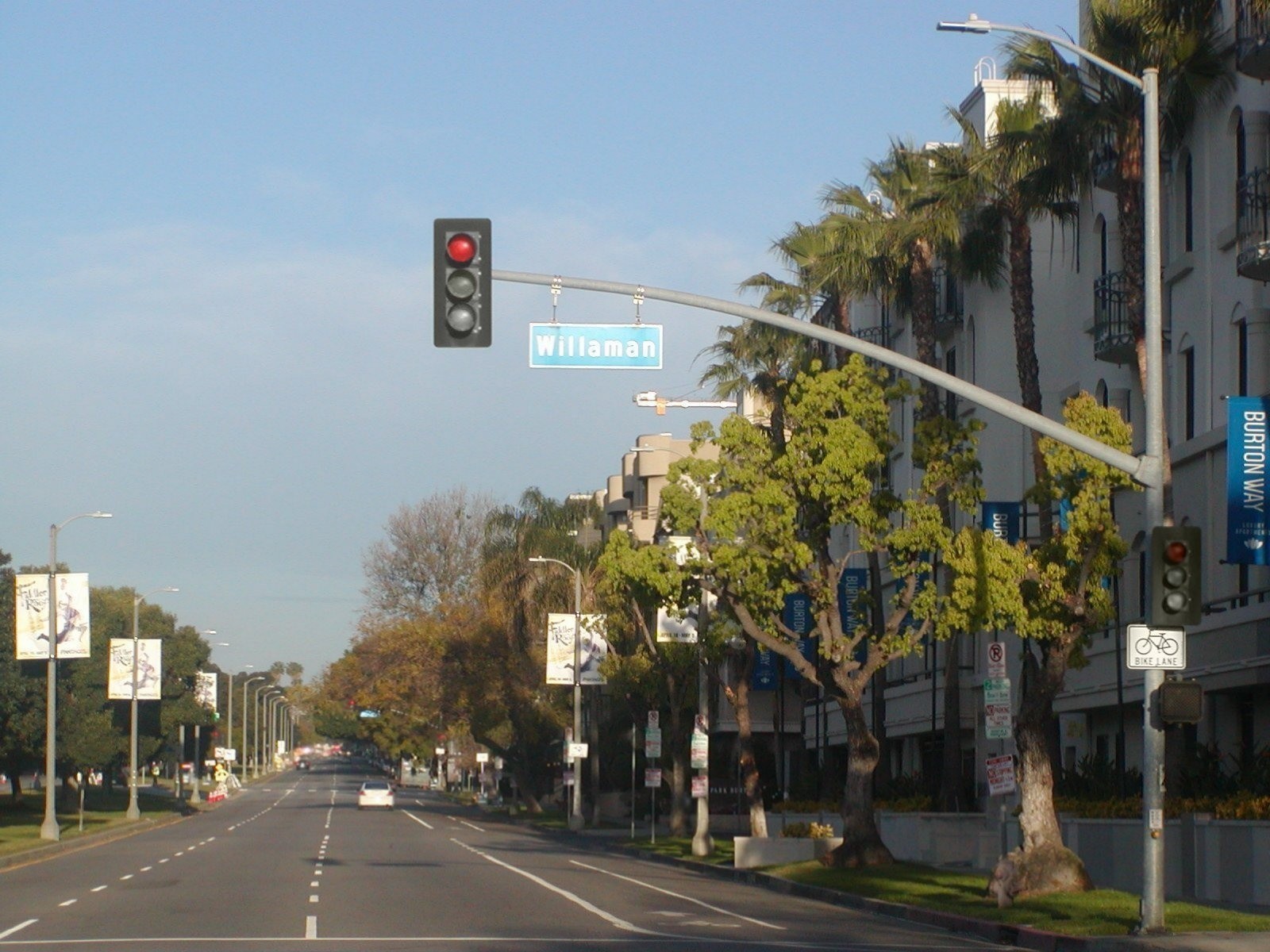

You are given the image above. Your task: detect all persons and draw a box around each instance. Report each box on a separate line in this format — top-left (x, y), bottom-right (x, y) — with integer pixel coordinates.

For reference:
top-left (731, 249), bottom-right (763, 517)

top-left (151, 762), bottom-right (161, 789)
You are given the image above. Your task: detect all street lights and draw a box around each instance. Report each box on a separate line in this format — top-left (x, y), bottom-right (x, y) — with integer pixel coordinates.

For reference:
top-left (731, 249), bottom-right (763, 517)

top-left (190, 642), bottom-right (231, 804)
top-left (254, 684), bottom-right (276, 780)
top-left (228, 664), bottom-right (253, 776)
top-left (39, 511), bottom-right (111, 842)
top-left (935, 17), bottom-right (1173, 936)
top-left (263, 690), bottom-right (306, 772)
top-left (241, 675), bottom-right (265, 784)
top-left (126, 586), bottom-right (180, 825)
top-left (173, 629), bottom-right (218, 798)
top-left (529, 556), bottom-right (587, 832)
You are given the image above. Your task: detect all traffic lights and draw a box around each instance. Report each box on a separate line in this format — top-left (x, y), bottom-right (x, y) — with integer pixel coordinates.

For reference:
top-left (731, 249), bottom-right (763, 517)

top-left (1147, 523), bottom-right (1203, 629)
top-left (1156, 679), bottom-right (1203, 722)
top-left (432, 218), bottom-right (493, 348)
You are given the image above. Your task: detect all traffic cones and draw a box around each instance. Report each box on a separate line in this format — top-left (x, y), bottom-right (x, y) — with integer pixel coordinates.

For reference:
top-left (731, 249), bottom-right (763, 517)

top-left (207, 789), bottom-right (225, 804)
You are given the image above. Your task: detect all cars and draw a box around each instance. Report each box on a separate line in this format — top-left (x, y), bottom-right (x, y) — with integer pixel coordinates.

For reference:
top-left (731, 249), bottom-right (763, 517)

top-left (296, 760), bottom-right (310, 771)
top-left (360, 782), bottom-right (396, 812)
